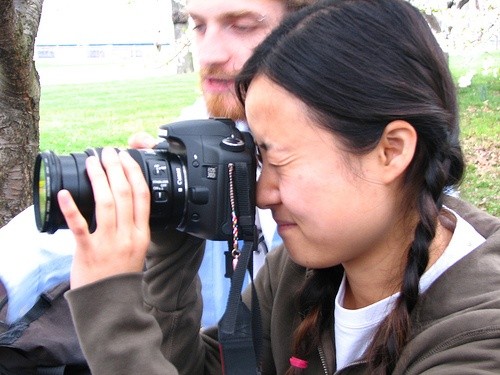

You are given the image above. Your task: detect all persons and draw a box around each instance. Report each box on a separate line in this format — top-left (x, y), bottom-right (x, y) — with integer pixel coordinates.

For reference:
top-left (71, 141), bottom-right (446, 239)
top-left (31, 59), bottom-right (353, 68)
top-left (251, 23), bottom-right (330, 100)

top-left (0, 0), bottom-right (284, 375)
top-left (55, 1), bottom-right (500, 375)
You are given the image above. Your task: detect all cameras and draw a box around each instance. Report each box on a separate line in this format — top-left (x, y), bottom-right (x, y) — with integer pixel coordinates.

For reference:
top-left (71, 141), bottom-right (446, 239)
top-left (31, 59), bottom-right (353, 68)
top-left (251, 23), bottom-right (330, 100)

top-left (34, 117), bottom-right (258, 252)
